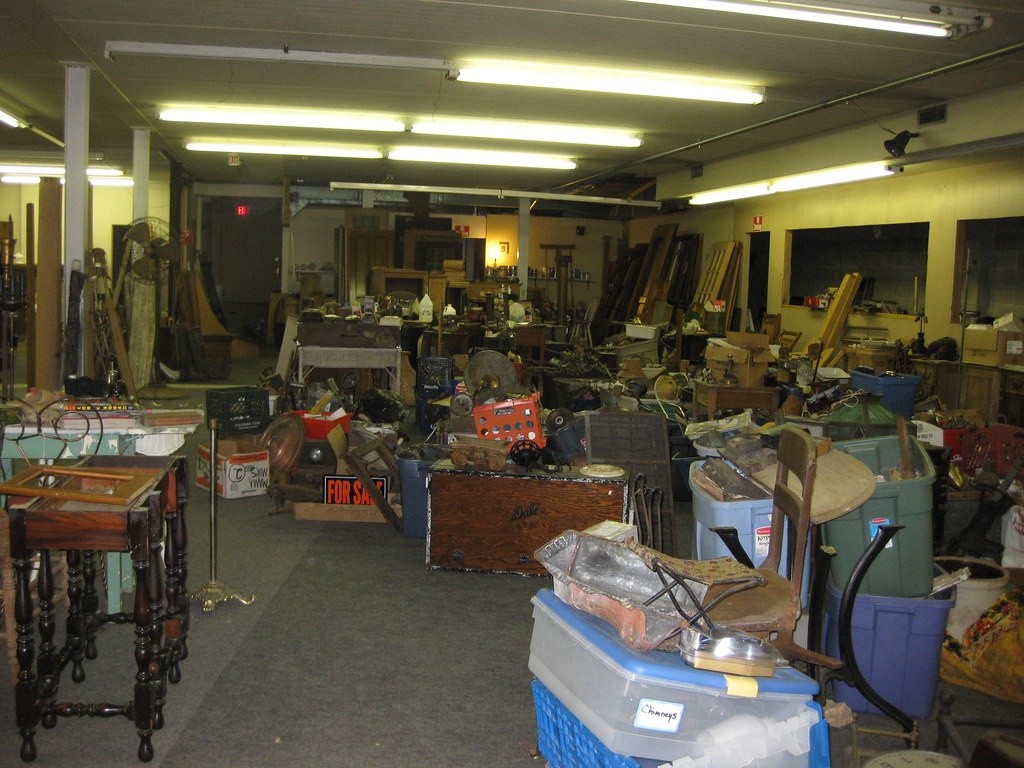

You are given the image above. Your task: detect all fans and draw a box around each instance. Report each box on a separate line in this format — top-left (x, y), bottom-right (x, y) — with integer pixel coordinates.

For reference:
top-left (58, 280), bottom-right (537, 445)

top-left (120, 214), bottom-right (186, 399)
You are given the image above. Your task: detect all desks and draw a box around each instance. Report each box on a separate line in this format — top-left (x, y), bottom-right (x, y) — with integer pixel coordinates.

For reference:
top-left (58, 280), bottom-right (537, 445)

top-left (296, 346), bottom-right (403, 395)
top-left (8, 446), bottom-right (192, 765)
top-left (692, 378), bottom-right (779, 422)
top-left (501, 322), bottom-right (546, 394)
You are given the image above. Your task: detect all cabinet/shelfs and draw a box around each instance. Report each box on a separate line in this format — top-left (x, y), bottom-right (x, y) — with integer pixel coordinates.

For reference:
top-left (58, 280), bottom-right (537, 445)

top-left (405, 228), bottom-right (466, 270)
top-left (678, 333), bottom-right (726, 364)
top-left (372, 265), bottom-right (428, 314)
top-left (465, 281), bottom-right (527, 318)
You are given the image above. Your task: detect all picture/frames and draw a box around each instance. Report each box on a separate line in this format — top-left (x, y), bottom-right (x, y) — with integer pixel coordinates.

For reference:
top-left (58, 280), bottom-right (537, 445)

top-left (0, 461), bottom-right (158, 507)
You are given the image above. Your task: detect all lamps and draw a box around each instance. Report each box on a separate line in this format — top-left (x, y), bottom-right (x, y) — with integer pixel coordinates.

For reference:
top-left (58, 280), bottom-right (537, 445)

top-left (450, 57), bottom-right (770, 109)
top-left (410, 113), bottom-right (647, 151)
top-left (0, 99), bottom-right (135, 188)
top-left (150, 102), bottom-right (407, 136)
top-left (686, 153), bottom-right (911, 208)
top-left (178, 132), bottom-right (384, 165)
top-left (102, 40), bottom-right (455, 93)
top-left (632, 0), bottom-right (996, 45)
top-left (387, 143), bottom-right (583, 174)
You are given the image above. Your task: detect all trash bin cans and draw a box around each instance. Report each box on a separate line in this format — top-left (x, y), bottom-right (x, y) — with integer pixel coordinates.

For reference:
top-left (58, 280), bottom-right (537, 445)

top-left (820, 433), bottom-right (936, 599)
top-left (820, 562), bottom-right (957, 718)
top-left (850, 366), bottom-right (922, 421)
top-left (394, 444), bottom-right (452, 538)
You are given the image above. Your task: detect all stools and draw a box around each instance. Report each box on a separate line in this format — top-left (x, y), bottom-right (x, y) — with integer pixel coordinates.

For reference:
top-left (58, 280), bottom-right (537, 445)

top-left (631, 541), bottom-right (762, 648)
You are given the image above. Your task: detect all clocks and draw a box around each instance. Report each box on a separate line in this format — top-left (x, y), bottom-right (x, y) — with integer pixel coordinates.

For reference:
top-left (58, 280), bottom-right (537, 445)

top-left (860, 750), bottom-right (974, 768)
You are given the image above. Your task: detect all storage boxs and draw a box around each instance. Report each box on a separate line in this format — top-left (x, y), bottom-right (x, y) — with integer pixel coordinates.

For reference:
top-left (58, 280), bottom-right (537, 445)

top-left (624, 321), bottom-right (657, 340)
top-left (554, 376), bottom-right (599, 410)
top-left (412, 391), bottom-right (448, 434)
top-left (963, 328), bottom-right (1024, 366)
top-left (612, 340), bottom-right (662, 364)
top-left (910, 412), bottom-right (987, 461)
top-left (819, 564), bottom-right (958, 718)
top-left (704, 309), bottom-right (727, 332)
top-left (204, 387), bottom-right (271, 436)
top-left (804, 296), bottom-right (830, 309)
top-left (426, 458), bottom-right (632, 580)
top-left (815, 366), bottom-right (850, 386)
top-left (484, 333), bottom-right (516, 350)
top-left (473, 395), bottom-right (546, 451)
top-left (535, 340), bottom-right (578, 362)
top-left (849, 367), bottom-right (922, 416)
top-left (204, 332), bottom-right (235, 362)
top-left (820, 430), bottom-right (935, 596)
top-left (525, 587), bottom-right (824, 759)
top-left (549, 417), bottom-right (584, 458)
top-left (704, 331), bottom-right (776, 387)
top-left (685, 458), bottom-right (810, 606)
top-left (296, 318), bottom-right (400, 348)
top-left (618, 394), bottom-right (682, 414)
top-left (416, 355), bottom-right (454, 394)
top-left (195, 432), bottom-right (268, 500)
top-left (397, 458), bottom-right (427, 538)
top-left (530, 675), bottom-right (641, 768)
top-left (290, 410), bottom-right (354, 440)
top-left (994, 312), bottom-right (1024, 331)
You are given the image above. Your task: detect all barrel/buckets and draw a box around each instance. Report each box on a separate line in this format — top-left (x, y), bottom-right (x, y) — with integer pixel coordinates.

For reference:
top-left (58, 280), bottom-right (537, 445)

top-left (933, 556), bottom-right (1009, 640)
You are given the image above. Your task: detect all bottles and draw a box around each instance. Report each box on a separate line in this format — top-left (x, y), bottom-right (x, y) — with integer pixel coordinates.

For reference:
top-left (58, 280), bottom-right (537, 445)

top-left (419, 294), bottom-right (433, 322)
top-left (402, 300), bottom-right (412, 316)
top-left (442, 304), bottom-right (456, 315)
top-left (509, 301), bottom-right (525, 322)
